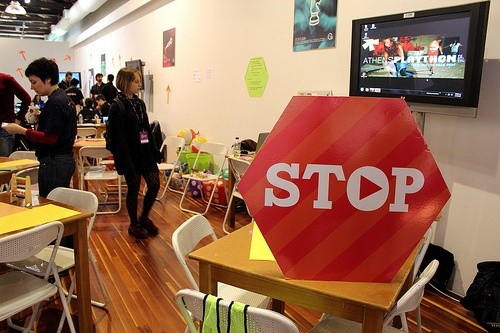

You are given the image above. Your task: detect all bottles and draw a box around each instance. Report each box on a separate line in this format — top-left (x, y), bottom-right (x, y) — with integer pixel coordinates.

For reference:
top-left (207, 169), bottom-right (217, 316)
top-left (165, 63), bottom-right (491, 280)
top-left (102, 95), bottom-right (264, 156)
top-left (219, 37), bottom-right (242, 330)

top-left (234, 137), bottom-right (240, 157)
top-left (79, 114), bottom-right (83, 124)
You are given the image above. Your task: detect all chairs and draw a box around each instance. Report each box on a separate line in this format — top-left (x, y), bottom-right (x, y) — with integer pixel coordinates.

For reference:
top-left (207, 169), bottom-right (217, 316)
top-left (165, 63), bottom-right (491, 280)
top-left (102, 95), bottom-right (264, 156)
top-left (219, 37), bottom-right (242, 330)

top-left (176, 288), bottom-right (301, 333)
top-left (143, 136), bottom-right (186, 201)
top-left (171, 214), bottom-right (273, 311)
top-left (222, 155), bottom-right (251, 235)
top-left (0, 128), bottom-right (128, 333)
top-left (307, 259), bottom-right (440, 333)
top-left (179, 143), bottom-right (230, 215)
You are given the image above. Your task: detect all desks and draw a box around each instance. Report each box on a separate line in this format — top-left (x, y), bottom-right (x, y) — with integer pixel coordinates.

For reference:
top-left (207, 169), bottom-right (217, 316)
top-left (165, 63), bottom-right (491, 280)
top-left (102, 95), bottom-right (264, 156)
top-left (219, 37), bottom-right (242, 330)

top-left (0, 191), bottom-right (95, 332)
top-left (72, 139), bottom-right (107, 189)
top-left (189, 223), bottom-right (425, 333)
top-left (0, 156), bottom-right (40, 187)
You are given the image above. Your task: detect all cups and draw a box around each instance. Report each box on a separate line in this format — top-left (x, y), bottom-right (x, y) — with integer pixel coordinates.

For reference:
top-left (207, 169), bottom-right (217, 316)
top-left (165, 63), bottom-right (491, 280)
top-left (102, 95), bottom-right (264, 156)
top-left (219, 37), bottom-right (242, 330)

top-left (96, 119), bottom-right (101, 124)
top-left (240, 151), bottom-right (248, 160)
top-left (28, 106), bottom-right (40, 123)
top-left (103, 117), bottom-right (108, 125)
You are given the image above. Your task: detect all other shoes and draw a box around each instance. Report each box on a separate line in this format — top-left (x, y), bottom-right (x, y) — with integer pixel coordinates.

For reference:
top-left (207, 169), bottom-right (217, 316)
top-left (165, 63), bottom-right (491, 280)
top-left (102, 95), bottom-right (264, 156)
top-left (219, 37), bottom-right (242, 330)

top-left (128, 224), bottom-right (148, 238)
top-left (139, 216), bottom-right (159, 234)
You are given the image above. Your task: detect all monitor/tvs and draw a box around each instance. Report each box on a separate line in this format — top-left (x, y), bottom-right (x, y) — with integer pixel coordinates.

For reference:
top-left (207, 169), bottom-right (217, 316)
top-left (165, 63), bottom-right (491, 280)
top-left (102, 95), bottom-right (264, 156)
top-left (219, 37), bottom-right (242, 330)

top-left (124, 59), bottom-right (144, 90)
top-left (57, 72), bottom-right (81, 88)
top-left (348, 1), bottom-right (491, 107)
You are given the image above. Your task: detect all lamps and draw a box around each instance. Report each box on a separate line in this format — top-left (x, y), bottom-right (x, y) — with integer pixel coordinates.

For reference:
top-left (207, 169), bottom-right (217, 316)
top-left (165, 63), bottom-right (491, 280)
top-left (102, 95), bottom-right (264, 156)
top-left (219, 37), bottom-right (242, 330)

top-left (4, 0), bottom-right (26, 15)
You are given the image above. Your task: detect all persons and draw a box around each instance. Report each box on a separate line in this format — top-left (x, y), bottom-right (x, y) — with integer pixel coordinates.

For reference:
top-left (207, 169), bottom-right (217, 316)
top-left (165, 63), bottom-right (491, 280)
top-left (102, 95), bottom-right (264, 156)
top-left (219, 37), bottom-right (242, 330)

top-left (1, 57), bottom-right (78, 246)
top-left (78, 98), bottom-right (100, 120)
top-left (32, 95), bottom-right (41, 106)
top-left (375, 37), bottom-right (417, 75)
top-left (445, 40), bottom-right (463, 60)
top-left (428, 38), bottom-right (443, 75)
top-left (65, 78), bottom-right (84, 106)
top-left (106, 69), bottom-right (160, 240)
top-left (58, 71), bottom-right (73, 90)
top-left (0, 73), bottom-right (31, 158)
top-left (101, 74), bottom-right (118, 104)
top-left (96, 94), bottom-right (111, 123)
top-left (90, 73), bottom-right (106, 101)
